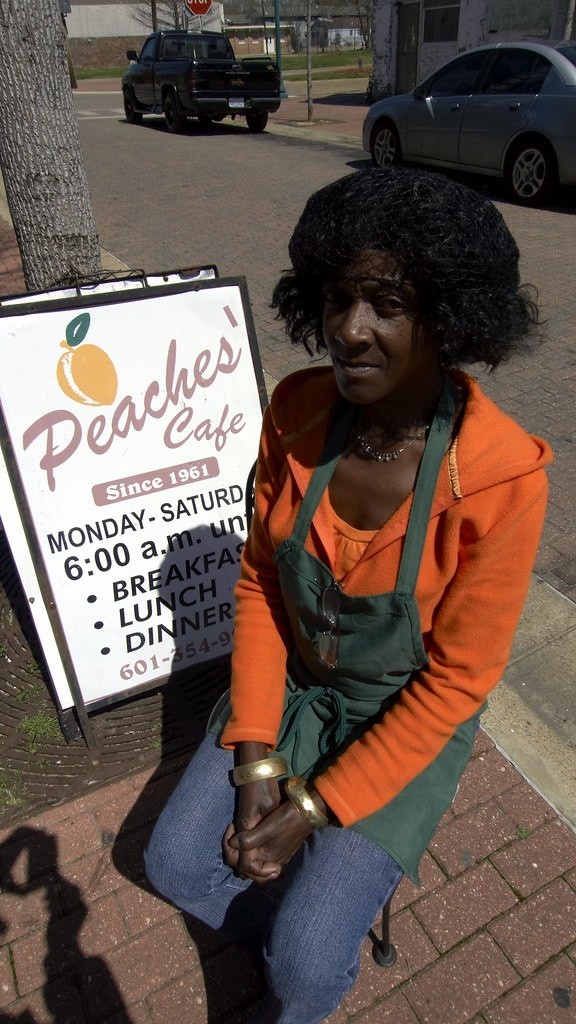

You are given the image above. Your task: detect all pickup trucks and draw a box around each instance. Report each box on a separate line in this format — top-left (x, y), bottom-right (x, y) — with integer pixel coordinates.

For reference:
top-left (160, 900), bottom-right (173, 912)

top-left (120, 29), bottom-right (282, 134)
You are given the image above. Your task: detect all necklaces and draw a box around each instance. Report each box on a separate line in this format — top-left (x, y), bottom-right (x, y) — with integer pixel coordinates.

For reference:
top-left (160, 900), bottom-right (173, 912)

top-left (351, 406), bottom-right (431, 462)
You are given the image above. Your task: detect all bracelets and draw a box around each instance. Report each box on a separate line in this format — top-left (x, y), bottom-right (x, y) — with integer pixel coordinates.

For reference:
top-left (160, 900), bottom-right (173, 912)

top-left (284, 775), bottom-right (328, 831)
top-left (230, 756), bottom-right (288, 785)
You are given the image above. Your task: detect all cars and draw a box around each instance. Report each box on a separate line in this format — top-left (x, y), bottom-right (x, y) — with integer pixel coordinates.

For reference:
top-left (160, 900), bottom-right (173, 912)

top-left (363, 39), bottom-right (576, 205)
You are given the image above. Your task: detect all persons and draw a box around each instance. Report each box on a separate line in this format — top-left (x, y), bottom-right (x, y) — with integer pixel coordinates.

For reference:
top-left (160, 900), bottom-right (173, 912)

top-left (138, 162), bottom-right (554, 1024)
